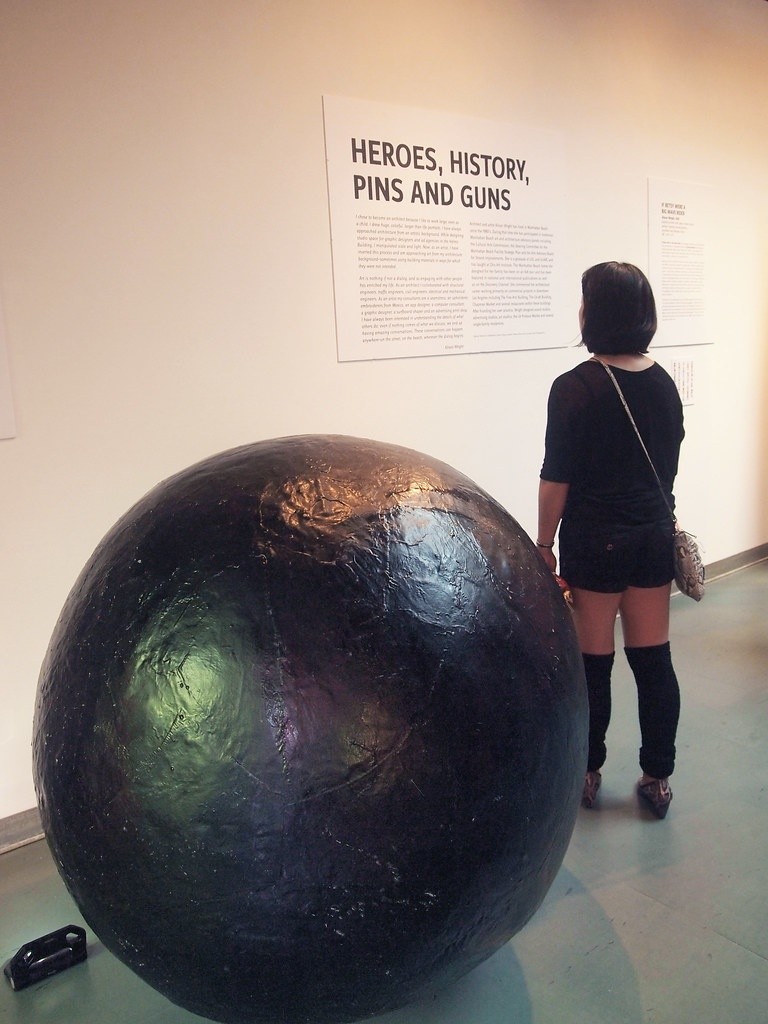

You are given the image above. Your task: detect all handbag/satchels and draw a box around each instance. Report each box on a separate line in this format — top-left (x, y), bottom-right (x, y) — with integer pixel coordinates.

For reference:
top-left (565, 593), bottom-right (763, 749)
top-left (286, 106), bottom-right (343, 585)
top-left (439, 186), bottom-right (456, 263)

top-left (673, 529), bottom-right (705, 602)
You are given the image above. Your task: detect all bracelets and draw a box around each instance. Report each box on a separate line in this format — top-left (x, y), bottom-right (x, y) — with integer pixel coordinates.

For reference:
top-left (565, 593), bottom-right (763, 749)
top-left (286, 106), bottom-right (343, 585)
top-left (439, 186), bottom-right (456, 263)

top-left (536, 540), bottom-right (554, 548)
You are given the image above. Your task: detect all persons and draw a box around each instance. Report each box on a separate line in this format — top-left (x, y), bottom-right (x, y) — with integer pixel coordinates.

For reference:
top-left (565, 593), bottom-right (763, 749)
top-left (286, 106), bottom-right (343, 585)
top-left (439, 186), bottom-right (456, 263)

top-left (535, 260), bottom-right (681, 819)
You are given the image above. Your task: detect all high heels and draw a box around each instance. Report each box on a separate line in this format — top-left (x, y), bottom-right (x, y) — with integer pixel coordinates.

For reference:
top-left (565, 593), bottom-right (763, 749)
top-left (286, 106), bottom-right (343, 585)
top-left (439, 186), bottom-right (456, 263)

top-left (638, 776), bottom-right (673, 819)
top-left (581, 772), bottom-right (601, 808)
